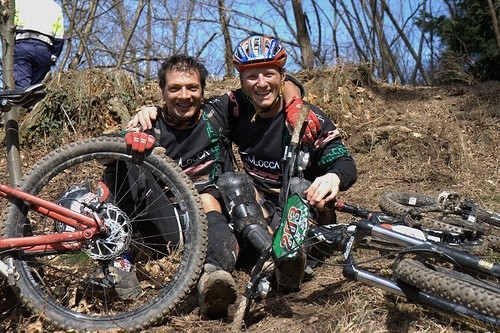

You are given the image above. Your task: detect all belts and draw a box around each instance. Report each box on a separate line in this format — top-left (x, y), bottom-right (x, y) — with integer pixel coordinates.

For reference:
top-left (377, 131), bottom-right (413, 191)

top-left (13, 30), bottom-right (54, 46)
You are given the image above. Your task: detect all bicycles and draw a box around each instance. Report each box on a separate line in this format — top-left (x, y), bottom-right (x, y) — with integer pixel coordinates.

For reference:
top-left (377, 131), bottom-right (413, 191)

top-left (229, 102), bottom-right (500, 333)
top-left (0, 83), bottom-right (209, 333)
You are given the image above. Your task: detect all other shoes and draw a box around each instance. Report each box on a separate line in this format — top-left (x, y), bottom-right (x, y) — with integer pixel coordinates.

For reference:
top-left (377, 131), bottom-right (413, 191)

top-left (102, 256), bottom-right (142, 302)
top-left (197, 269), bottom-right (238, 320)
top-left (274, 243), bottom-right (307, 293)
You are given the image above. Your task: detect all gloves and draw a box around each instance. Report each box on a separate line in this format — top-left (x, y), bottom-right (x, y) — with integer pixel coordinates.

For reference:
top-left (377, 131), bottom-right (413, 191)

top-left (124, 132), bottom-right (157, 153)
top-left (285, 96), bottom-right (321, 142)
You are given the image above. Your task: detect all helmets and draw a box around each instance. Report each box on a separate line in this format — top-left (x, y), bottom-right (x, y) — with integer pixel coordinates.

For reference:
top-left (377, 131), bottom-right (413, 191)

top-left (231, 34), bottom-right (287, 71)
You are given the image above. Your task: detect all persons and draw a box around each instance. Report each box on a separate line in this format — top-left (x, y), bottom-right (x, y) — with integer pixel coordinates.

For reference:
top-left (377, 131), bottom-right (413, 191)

top-left (101, 54), bottom-right (321, 325)
top-left (126, 33), bottom-right (357, 299)
top-left (13, 0), bottom-right (64, 90)
